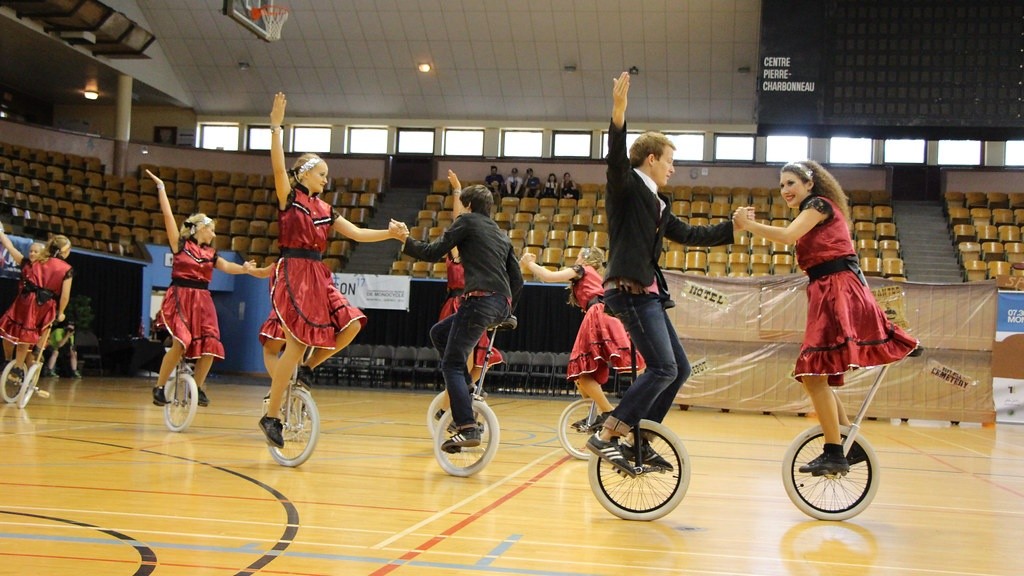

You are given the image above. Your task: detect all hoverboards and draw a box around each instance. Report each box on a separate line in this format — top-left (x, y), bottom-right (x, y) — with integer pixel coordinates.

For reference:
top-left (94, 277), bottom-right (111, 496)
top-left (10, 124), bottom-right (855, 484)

top-left (558, 397), bottom-right (603, 461)
top-left (782, 346), bottom-right (923, 521)
top-left (262, 363), bottom-right (320, 469)
top-left (589, 301), bottom-right (690, 522)
top-left (0, 314), bottom-right (58, 409)
top-left (427, 315), bottom-right (518, 477)
top-left (153, 358), bottom-right (208, 433)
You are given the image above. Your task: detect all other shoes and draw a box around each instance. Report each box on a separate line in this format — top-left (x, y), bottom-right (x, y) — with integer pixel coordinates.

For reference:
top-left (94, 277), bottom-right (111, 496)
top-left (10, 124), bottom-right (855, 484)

top-left (8, 367), bottom-right (24, 383)
top-left (564, 193), bottom-right (574, 199)
top-left (46, 370), bottom-right (59, 378)
top-left (72, 370), bottom-right (82, 379)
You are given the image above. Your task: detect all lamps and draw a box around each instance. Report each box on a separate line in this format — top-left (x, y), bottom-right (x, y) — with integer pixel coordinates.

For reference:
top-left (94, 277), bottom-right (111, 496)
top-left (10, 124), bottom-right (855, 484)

top-left (239, 61), bottom-right (251, 70)
top-left (628, 67), bottom-right (639, 74)
top-left (418, 63), bottom-right (430, 73)
top-left (84, 68), bottom-right (101, 99)
top-left (565, 66), bottom-right (576, 73)
top-left (738, 68), bottom-right (752, 73)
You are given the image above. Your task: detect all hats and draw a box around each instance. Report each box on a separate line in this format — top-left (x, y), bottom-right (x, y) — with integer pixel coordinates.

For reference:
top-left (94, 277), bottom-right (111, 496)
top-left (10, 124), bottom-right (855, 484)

top-left (511, 168), bottom-right (518, 173)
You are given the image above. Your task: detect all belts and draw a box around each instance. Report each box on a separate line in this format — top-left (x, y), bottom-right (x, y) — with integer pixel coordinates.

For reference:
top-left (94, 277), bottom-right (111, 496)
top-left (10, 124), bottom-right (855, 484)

top-left (461, 290), bottom-right (512, 309)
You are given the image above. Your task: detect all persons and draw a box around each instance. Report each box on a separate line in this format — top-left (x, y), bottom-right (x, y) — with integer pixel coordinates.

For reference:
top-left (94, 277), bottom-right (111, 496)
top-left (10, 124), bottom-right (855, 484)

top-left (733, 159), bottom-right (919, 473)
top-left (586, 71), bottom-right (756, 478)
top-left (485, 165), bottom-right (582, 200)
top-left (521, 246), bottom-right (646, 433)
top-left (43, 321), bottom-right (82, 379)
top-left (0, 222), bottom-right (73, 376)
top-left (145, 169), bottom-right (256, 406)
top-left (243, 91), bottom-right (409, 448)
top-left (389, 169), bottom-right (536, 453)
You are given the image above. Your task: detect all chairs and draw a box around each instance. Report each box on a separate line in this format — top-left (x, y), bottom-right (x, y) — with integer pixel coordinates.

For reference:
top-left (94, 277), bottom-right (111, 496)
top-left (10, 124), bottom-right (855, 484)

top-left (945, 190), bottom-right (1024, 281)
top-left (301, 347), bottom-right (634, 398)
top-left (0, 145), bottom-right (909, 277)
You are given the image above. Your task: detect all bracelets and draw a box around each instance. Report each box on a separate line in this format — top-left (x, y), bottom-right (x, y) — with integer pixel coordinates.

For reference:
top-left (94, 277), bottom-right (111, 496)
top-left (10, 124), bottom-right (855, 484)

top-left (453, 189), bottom-right (461, 196)
top-left (156, 184), bottom-right (165, 190)
top-left (270, 126), bottom-right (282, 133)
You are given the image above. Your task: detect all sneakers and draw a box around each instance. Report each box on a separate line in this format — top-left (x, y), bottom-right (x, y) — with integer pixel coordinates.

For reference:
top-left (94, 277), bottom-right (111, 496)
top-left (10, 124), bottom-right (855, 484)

top-left (571, 415), bottom-right (590, 429)
top-left (258, 414), bottom-right (284, 448)
top-left (297, 365), bottom-right (314, 390)
top-left (436, 386), bottom-right (478, 417)
top-left (844, 443), bottom-right (867, 465)
top-left (585, 432), bottom-right (637, 480)
top-left (441, 427), bottom-right (481, 451)
top-left (620, 439), bottom-right (674, 472)
top-left (590, 411), bottom-right (615, 431)
top-left (153, 385), bottom-right (167, 403)
top-left (799, 449), bottom-right (850, 473)
top-left (189, 386), bottom-right (209, 407)
